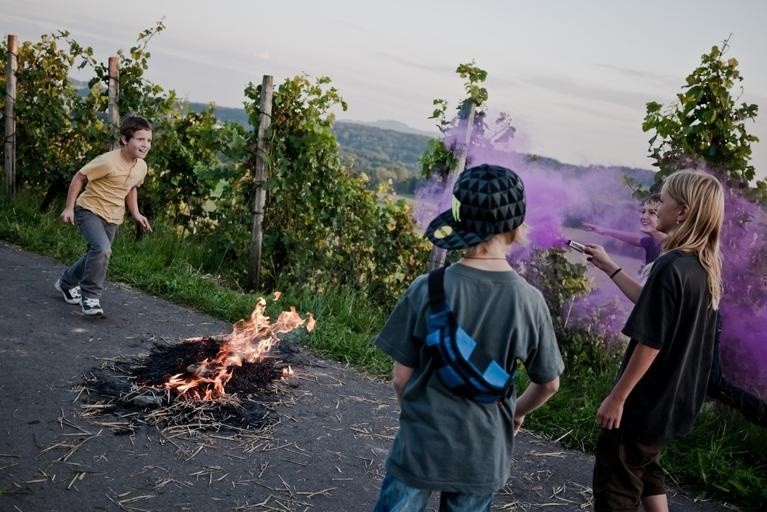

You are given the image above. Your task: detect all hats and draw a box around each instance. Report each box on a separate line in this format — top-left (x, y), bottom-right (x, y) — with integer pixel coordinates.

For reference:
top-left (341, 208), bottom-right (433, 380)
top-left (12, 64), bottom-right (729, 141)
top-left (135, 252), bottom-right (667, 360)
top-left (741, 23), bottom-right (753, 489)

top-left (427, 164), bottom-right (526, 249)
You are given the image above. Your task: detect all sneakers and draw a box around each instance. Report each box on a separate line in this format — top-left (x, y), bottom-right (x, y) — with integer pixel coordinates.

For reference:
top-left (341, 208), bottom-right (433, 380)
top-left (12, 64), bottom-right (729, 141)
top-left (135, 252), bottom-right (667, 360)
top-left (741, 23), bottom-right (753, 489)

top-left (54, 276), bottom-right (103, 316)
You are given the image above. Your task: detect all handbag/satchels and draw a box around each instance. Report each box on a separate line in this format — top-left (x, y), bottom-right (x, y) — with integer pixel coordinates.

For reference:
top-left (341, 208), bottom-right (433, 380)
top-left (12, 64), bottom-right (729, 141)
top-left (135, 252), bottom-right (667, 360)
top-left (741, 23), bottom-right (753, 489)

top-left (425, 262), bottom-right (512, 404)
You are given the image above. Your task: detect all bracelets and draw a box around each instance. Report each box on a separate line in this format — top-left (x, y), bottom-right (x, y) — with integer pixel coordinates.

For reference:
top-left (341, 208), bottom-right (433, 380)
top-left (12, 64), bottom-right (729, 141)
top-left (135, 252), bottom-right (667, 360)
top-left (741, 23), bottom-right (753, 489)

top-left (610, 268), bottom-right (622, 279)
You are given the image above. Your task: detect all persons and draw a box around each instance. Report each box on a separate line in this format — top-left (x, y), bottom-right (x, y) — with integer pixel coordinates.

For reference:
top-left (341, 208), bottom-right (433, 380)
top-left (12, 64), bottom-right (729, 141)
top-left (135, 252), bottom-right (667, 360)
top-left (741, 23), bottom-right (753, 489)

top-left (374, 163), bottom-right (565, 512)
top-left (583, 171), bottom-right (725, 511)
top-left (581, 195), bottom-right (670, 265)
top-left (54, 116), bottom-right (153, 316)
